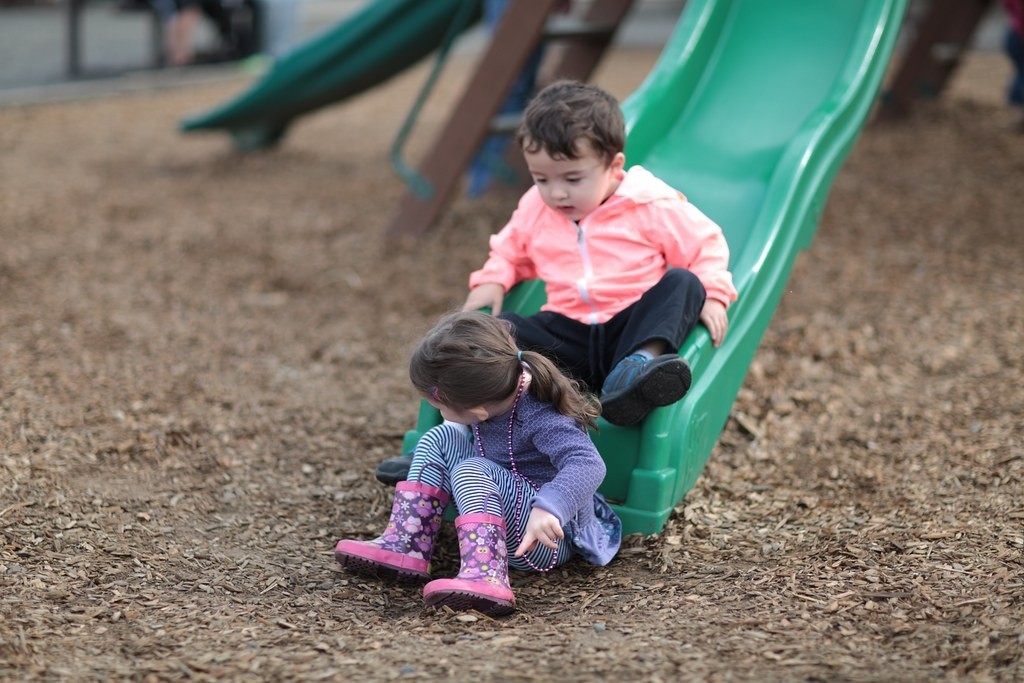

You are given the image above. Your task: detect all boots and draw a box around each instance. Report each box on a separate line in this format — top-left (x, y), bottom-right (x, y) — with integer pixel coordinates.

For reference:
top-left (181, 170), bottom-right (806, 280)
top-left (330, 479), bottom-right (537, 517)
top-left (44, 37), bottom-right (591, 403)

top-left (421, 513), bottom-right (517, 617)
top-left (335, 482), bottom-right (448, 587)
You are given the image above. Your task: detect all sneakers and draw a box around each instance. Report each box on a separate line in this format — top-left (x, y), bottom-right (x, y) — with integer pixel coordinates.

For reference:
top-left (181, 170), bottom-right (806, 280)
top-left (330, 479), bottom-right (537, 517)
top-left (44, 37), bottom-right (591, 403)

top-left (378, 450), bottom-right (414, 482)
top-left (602, 353), bottom-right (691, 426)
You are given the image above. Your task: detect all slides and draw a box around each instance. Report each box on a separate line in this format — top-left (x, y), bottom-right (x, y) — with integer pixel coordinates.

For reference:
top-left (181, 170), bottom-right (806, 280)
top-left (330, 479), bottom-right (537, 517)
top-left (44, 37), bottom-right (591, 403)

top-left (176, 1), bottom-right (486, 155)
top-left (378, 0), bottom-right (912, 536)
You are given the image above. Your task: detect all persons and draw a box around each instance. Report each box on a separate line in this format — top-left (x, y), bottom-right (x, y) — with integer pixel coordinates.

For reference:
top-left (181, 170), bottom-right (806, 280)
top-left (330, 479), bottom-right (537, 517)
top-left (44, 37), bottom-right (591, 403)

top-left (333, 311), bottom-right (607, 617)
top-left (460, 79), bottom-right (738, 426)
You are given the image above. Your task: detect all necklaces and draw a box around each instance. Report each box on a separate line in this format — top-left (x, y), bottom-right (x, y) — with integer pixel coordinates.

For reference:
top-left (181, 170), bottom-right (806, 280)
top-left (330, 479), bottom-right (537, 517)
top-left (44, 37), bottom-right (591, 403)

top-left (475, 367), bottom-right (559, 572)
